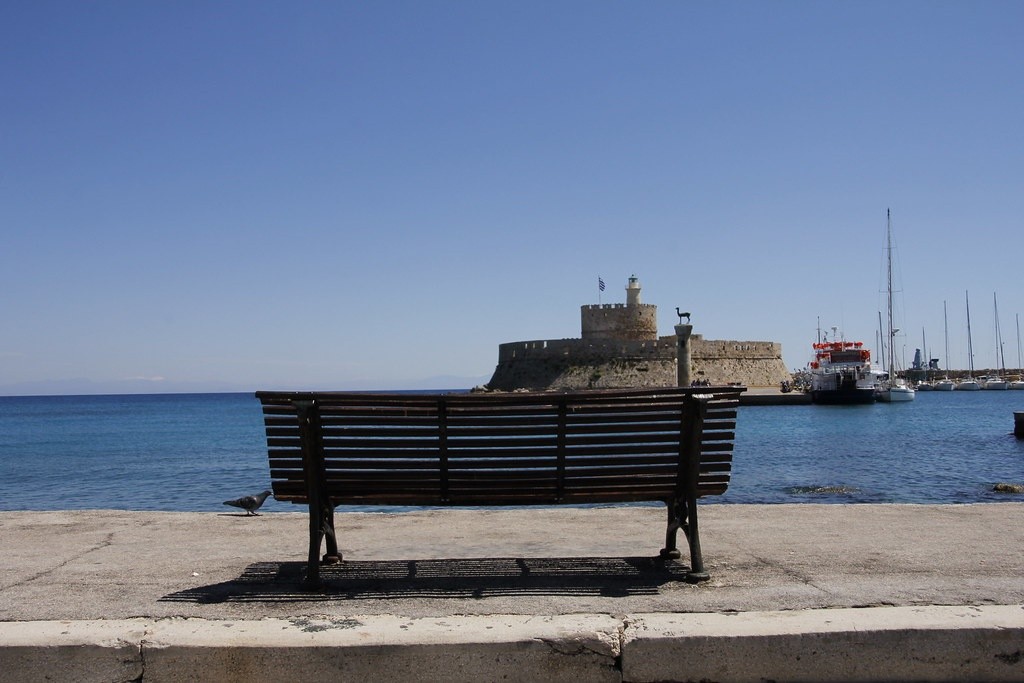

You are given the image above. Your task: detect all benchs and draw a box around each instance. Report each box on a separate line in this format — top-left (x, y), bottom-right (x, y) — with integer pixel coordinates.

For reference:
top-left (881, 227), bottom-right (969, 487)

top-left (254, 385), bottom-right (748, 593)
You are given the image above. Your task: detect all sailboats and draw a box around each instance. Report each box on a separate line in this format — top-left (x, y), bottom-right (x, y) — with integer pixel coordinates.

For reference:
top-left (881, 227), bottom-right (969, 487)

top-left (982, 292), bottom-right (1009, 391)
top-left (933, 301), bottom-right (955, 391)
top-left (917, 325), bottom-right (934, 391)
top-left (1010, 313), bottom-right (1024, 390)
top-left (956, 290), bottom-right (982, 391)
top-left (871, 207), bottom-right (917, 402)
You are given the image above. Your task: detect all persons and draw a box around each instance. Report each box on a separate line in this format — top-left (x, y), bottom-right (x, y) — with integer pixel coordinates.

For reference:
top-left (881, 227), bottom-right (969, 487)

top-left (691, 378), bottom-right (711, 387)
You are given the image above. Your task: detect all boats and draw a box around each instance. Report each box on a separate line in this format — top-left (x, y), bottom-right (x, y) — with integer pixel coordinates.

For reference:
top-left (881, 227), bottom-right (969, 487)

top-left (807, 316), bottom-right (877, 405)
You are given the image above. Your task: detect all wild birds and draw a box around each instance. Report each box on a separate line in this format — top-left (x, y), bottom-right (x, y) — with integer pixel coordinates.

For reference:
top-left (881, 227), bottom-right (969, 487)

top-left (222, 489), bottom-right (275, 517)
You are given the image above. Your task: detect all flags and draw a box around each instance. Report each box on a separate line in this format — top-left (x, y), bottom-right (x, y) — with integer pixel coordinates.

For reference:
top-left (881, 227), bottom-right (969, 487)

top-left (599, 278), bottom-right (605, 291)
